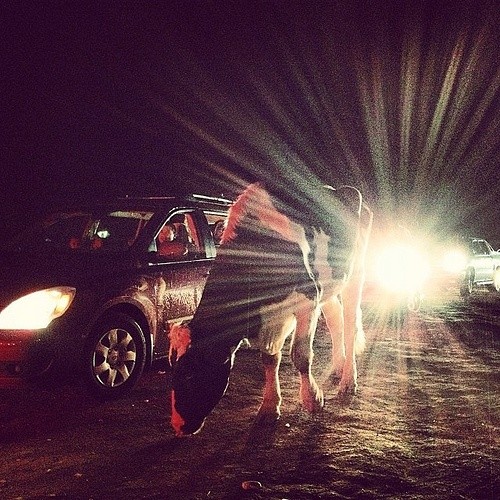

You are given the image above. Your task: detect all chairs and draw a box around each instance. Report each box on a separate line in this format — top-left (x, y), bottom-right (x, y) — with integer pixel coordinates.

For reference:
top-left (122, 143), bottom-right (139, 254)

top-left (175, 224), bottom-right (198, 261)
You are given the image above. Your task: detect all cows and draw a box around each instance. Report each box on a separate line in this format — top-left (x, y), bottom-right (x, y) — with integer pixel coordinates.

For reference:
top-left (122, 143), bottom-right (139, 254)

top-left (166, 178), bottom-right (375, 438)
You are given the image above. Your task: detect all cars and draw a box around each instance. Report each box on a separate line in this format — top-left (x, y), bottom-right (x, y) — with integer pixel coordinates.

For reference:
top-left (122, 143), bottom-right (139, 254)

top-left (0, 196), bottom-right (234, 389)
top-left (413, 235), bottom-right (500, 301)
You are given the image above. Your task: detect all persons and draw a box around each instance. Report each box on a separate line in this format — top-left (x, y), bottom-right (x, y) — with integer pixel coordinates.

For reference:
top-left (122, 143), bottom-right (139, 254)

top-left (156, 221), bottom-right (198, 256)
